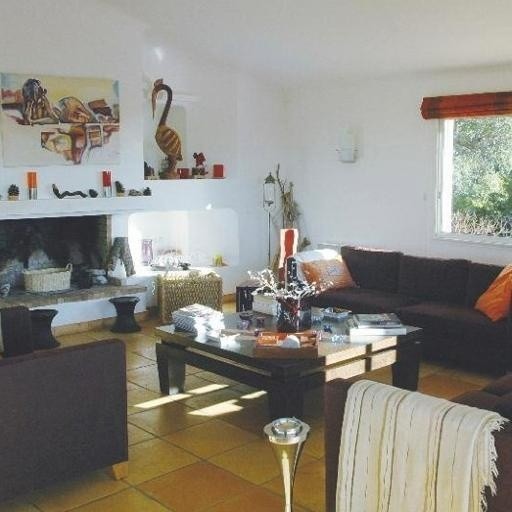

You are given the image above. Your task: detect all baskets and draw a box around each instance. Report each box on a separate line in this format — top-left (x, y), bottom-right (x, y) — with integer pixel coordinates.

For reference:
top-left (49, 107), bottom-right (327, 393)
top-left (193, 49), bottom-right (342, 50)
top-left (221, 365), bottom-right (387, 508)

top-left (157, 271), bottom-right (223, 325)
top-left (22, 264), bottom-right (71, 293)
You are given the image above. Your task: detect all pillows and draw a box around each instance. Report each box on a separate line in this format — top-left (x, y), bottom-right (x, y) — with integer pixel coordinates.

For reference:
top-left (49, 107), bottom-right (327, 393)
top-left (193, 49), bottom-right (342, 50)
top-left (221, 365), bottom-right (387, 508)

top-left (475, 264), bottom-right (512, 322)
top-left (1, 306), bottom-right (31, 356)
top-left (285, 248), bottom-right (359, 299)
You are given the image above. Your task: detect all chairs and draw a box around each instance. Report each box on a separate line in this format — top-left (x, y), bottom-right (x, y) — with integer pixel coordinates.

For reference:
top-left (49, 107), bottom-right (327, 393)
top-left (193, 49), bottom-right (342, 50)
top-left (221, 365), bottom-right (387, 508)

top-left (1, 302), bottom-right (129, 501)
top-left (325, 375), bottom-right (512, 512)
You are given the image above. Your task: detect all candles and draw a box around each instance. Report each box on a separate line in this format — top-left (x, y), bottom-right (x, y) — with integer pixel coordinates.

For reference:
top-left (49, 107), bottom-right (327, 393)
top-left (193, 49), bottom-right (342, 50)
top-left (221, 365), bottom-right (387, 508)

top-left (103, 171), bottom-right (111, 185)
top-left (213, 165), bottom-right (223, 176)
top-left (28, 172), bottom-right (37, 188)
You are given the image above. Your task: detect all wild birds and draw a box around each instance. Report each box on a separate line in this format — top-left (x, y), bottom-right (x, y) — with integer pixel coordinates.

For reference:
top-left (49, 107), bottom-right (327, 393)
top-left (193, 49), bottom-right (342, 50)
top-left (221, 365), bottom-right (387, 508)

top-left (152, 84), bottom-right (183, 172)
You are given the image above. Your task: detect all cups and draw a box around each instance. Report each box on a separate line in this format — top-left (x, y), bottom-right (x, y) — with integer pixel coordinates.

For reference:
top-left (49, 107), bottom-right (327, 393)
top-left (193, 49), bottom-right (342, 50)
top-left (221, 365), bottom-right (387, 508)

top-left (239, 303), bottom-right (254, 320)
top-left (256, 317), bottom-right (266, 330)
top-left (213, 164), bottom-right (224, 177)
top-left (311, 313), bottom-right (350, 343)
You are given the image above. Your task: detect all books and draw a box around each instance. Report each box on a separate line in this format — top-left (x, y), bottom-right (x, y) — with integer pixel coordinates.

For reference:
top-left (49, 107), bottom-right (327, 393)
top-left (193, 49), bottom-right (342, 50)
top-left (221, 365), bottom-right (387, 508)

top-left (352, 312), bottom-right (401, 328)
top-left (344, 314), bottom-right (406, 336)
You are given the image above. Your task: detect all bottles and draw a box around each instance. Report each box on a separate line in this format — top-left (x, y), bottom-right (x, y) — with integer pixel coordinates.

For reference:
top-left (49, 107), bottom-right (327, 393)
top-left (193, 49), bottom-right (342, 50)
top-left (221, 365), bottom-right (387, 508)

top-left (141, 239), bottom-right (153, 263)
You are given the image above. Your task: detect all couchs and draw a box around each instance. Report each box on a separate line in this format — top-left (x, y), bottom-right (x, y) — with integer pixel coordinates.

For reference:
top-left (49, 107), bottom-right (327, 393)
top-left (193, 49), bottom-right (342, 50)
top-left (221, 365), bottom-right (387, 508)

top-left (284, 245), bottom-right (512, 376)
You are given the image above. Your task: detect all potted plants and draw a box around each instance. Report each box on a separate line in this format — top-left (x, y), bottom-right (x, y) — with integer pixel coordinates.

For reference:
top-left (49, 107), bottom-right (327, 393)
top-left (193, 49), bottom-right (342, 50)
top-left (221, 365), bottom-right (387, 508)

top-left (8, 184), bottom-right (20, 200)
top-left (115, 181), bottom-right (126, 197)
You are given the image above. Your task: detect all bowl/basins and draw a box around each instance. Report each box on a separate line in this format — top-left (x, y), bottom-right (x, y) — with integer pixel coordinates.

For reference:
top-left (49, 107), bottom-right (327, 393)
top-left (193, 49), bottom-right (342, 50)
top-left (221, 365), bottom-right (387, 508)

top-left (176, 167), bottom-right (189, 178)
